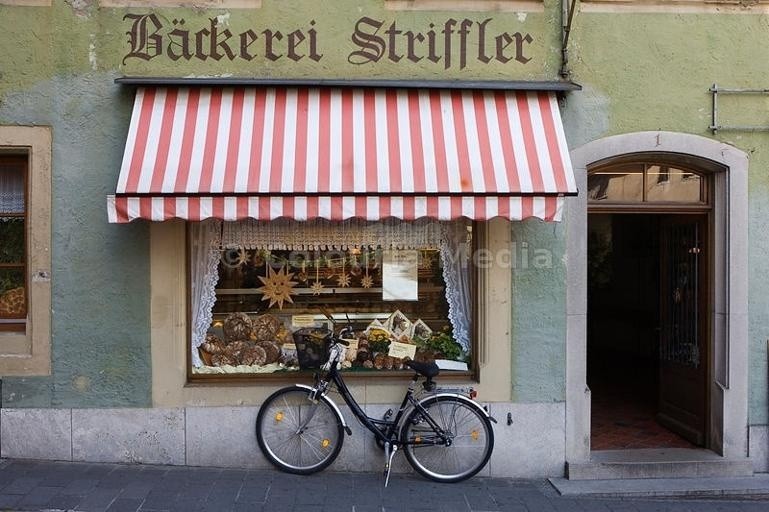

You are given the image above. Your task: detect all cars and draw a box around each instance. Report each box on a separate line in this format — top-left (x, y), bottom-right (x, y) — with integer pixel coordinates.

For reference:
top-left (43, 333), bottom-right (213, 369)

top-left (291, 313), bottom-right (391, 340)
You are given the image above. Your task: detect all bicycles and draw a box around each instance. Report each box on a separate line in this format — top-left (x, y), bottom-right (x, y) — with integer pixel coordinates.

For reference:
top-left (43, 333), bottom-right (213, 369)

top-left (252, 319), bottom-right (498, 487)
